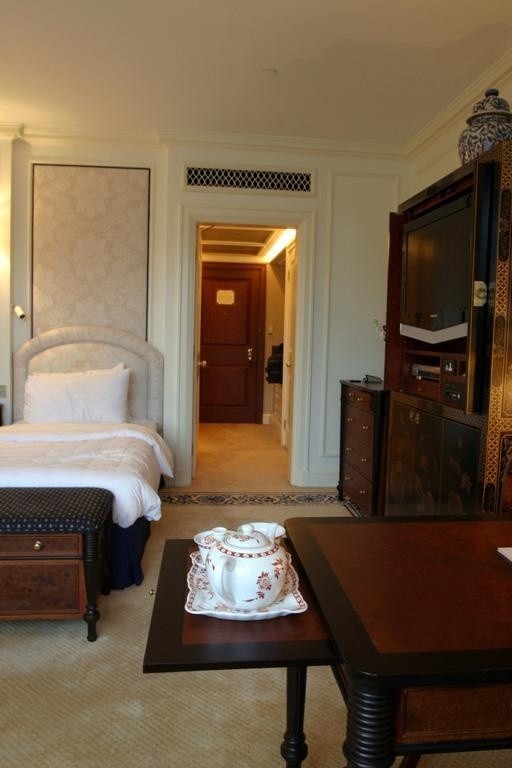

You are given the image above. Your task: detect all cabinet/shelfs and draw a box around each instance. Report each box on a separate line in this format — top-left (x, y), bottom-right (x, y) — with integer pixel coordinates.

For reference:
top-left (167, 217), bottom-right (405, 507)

top-left (337, 380), bottom-right (385, 518)
top-left (391, 173), bottom-right (509, 518)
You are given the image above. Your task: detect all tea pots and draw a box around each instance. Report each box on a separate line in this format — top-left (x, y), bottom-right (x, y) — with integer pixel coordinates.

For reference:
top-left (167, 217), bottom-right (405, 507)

top-left (203, 521), bottom-right (290, 612)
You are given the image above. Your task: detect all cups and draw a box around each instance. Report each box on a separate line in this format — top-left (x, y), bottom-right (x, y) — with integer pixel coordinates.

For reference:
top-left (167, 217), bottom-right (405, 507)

top-left (192, 525), bottom-right (235, 549)
top-left (236, 520), bottom-right (287, 540)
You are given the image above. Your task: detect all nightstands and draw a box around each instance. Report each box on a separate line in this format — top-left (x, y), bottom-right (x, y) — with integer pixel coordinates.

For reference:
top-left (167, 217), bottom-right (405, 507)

top-left (2, 487), bottom-right (117, 643)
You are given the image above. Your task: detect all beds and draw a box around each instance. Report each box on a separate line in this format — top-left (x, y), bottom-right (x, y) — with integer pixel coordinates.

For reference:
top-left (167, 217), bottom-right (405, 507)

top-left (0, 323), bottom-right (175, 590)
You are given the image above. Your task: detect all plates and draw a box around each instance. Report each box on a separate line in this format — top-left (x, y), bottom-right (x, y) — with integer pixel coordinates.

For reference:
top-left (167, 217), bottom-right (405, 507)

top-left (183, 549), bottom-right (309, 623)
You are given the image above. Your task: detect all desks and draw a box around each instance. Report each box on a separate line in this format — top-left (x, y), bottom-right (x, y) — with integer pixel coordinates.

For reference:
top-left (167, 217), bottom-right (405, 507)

top-left (142, 515), bottom-right (510, 767)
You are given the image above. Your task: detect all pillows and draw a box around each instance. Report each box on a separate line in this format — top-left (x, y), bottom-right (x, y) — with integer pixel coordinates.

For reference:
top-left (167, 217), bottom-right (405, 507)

top-left (23, 369), bottom-right (130, 423)
